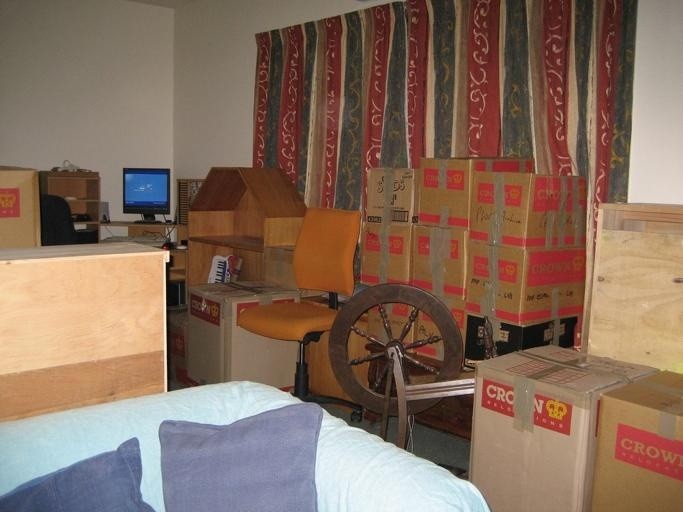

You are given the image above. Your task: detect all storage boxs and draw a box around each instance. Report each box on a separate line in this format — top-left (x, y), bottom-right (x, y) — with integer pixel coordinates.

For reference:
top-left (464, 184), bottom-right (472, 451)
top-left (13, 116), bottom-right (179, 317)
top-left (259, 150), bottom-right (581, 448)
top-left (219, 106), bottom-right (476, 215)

top-left (361, 220), bottom-right (410, 290)
top-left (365, 167), bottom-right (418, 225)
top-left (410, 292), bottom-right (466, 368)
top-left (464, 310), bottom-right (576, 364)
top-left (411, 225), bottom-right (469, 301)
top-left (587, 370), bottom-right (683, 511)
top-left (363, 291), bottom-right (414, 356)
top-left (187, 285), bottom-right (297, 395)
top-left (471, 169), bottom-right (587, 249)
top-left (467, 240), bottom-right (583, 324)
top-left (470, 342), bottom-right (659, 512)
top-left (418, 159), bottom-right (472, 232)
top-left (0, 167), bottom-right (40, 247)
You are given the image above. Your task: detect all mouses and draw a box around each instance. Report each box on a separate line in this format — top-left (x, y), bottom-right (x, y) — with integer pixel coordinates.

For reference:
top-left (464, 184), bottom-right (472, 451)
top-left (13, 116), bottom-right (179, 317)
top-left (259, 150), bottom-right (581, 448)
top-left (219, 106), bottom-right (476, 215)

top-left (162, 243), bottom-right (174, 250)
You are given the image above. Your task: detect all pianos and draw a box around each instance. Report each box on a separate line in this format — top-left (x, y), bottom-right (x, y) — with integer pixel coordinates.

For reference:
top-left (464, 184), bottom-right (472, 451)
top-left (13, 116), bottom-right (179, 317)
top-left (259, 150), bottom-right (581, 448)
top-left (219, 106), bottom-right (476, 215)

top-left (207, 255), bottom-right (233, 283)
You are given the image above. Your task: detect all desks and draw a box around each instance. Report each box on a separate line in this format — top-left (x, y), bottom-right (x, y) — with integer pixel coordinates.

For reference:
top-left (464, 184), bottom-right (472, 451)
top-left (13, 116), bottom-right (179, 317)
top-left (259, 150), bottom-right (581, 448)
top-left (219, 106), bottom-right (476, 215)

top-left (99, 221), bottom-right (188, 306)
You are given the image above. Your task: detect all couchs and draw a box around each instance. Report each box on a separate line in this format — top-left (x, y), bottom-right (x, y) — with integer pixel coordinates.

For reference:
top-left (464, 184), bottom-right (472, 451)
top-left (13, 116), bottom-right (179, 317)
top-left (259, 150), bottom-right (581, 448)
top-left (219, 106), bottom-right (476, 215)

top-left (0, 379), bottom-right (488, 512)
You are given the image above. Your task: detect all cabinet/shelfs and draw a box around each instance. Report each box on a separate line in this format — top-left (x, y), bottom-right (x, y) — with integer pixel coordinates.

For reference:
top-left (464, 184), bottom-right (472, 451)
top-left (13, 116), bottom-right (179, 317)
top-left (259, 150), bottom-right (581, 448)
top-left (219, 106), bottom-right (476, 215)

top-left (38, 171), bottom-right (100, 244)
top-left (183, 166), bottom-right (304, 287)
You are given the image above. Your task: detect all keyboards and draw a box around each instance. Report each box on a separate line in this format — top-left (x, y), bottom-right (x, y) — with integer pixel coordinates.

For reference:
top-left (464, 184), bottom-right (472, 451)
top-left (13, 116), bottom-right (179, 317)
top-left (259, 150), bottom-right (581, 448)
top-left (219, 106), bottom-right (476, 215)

top-left (103, 236), bottom-right (163, 246)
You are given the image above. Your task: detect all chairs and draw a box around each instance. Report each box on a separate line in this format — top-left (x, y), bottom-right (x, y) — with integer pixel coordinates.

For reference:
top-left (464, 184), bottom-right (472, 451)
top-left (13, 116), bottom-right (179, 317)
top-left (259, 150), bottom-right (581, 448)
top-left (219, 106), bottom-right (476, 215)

top-left (239, 209), bottom-right (364, 433)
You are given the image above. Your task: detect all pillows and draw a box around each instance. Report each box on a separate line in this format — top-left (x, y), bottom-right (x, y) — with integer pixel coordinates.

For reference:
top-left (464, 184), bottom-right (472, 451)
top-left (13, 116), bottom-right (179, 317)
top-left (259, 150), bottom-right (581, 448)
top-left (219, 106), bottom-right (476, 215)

top-left (0, 438), bottom-right (155, 512)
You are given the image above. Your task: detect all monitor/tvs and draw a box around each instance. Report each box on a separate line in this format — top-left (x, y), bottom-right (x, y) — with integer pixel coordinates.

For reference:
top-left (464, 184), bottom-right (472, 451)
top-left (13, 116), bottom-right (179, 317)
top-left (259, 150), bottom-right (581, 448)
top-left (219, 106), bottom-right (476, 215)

top-left (122, 168), bottom-right (170, 223)
